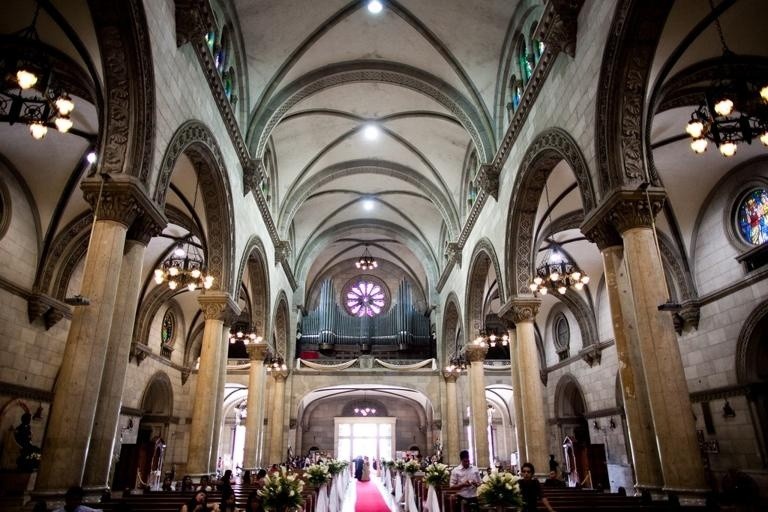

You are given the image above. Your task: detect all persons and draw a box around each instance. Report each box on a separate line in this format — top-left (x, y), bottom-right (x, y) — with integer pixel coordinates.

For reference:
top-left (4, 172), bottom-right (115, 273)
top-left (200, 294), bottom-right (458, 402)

top-left (163, 470), bottom-right (234, 512)
top-left (52, 485), bottom-right (103, 512)
top-left (405, 450), bottom-right (556, 512)
top-left (548, 454), bottom-right (558, 472)
top-left (243, 455), bottom-right (323, 489)
top-left (543, 471), bottom-right (566, 488)
top-left (352, 454), bottom-right (370, 482)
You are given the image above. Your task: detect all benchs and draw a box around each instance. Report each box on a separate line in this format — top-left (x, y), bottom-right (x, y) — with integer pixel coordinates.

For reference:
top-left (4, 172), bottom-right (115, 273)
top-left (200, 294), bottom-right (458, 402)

top-left (30, 468), bottom-right (336, 512)
top-left (408, 465), bottom-right (714, 512)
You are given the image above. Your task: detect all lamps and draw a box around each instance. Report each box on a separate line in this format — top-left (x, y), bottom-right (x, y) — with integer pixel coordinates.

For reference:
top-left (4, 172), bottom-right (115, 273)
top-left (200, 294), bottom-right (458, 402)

top-left (473, 258), bottom-right (511, 349)
top-left (528, 168), bottom-right (590, 297)
top-left (446, 325), bottom-right (472, 373)
top-left (151, 159), bottom-right (216, 294)
top-left (0, 51), bottom-right (74, 140)
top-left (684, 2), bottom-right (768, 160)
top-left (351, 389), bottom-right (378, 417)
top-left (355, 238), bottom-right (379, 273)
